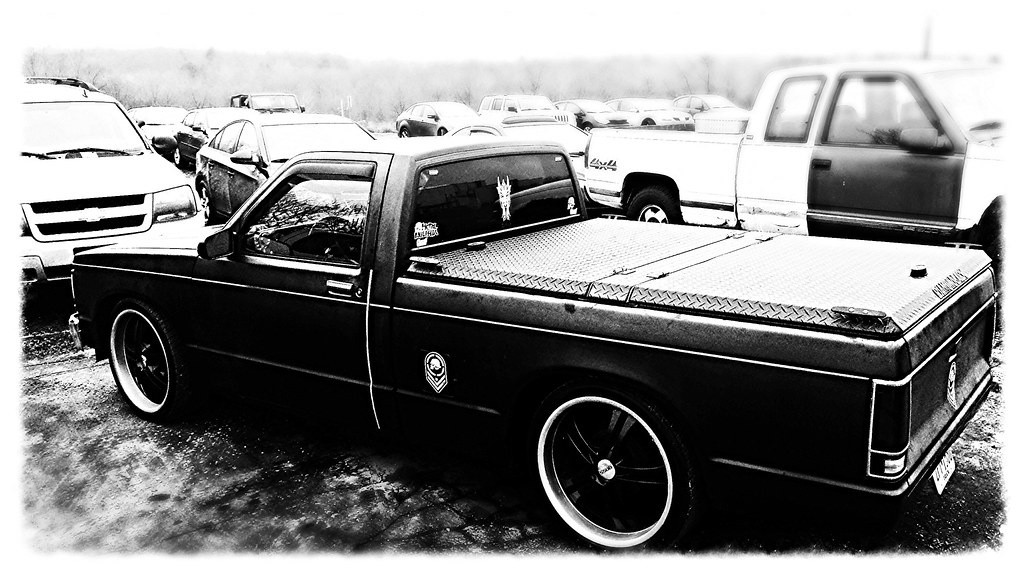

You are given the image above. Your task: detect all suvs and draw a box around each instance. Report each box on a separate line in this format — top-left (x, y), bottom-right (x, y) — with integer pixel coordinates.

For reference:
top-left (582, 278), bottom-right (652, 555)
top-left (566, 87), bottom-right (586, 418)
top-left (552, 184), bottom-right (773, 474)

top-left (21, 72), bottom-right (204, 326)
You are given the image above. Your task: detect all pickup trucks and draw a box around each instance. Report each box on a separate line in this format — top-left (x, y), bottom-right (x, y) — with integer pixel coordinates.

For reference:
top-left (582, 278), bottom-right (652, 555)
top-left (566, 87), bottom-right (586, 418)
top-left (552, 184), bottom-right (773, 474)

top-left (70, 134), bottom-right (998, 555)
top-left (582, 57), bottom-right (1013, 272)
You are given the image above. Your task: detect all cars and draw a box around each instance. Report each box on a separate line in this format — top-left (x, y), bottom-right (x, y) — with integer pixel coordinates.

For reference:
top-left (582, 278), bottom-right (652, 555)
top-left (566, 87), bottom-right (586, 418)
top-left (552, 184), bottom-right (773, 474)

top-left (128, 93), bottom-right (756, 225)
